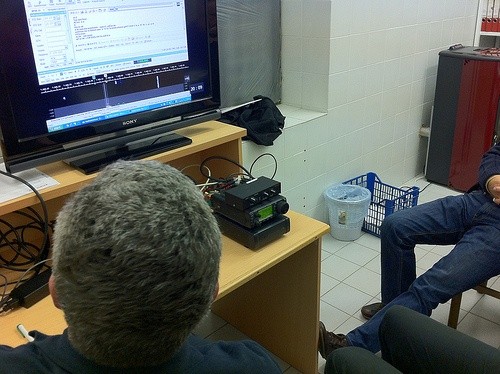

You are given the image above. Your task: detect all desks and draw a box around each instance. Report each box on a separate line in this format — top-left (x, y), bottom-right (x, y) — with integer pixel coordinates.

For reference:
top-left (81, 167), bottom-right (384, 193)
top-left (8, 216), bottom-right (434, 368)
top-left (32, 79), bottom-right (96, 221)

top-left (424, 46), bottom-right (500, 192)
top-left (0, 206), bottom-right (330, 374)
top-left (0, 118), bottom-right (248, 217)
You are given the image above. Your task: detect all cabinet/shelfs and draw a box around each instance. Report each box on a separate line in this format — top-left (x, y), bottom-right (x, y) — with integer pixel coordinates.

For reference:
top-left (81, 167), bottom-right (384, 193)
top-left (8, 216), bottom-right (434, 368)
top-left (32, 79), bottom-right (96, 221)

top-left (473, 0), bottom-right (500, 47)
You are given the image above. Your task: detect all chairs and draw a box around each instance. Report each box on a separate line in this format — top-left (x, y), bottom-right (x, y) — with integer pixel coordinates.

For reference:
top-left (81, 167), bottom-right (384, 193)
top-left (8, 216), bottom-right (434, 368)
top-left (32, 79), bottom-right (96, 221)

top-left (447, 280), bottom-right (500, 331)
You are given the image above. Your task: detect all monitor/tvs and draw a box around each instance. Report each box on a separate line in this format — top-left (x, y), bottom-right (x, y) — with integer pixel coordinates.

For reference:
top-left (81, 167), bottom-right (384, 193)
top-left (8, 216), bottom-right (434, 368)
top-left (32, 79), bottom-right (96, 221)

top-left (0, 0), bottom-right (222, 173)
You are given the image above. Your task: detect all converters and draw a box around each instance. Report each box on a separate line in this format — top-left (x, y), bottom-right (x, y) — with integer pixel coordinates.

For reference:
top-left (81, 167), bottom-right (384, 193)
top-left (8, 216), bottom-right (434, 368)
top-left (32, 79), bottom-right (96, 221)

top-left (13, 268), bottom-right (51, 309)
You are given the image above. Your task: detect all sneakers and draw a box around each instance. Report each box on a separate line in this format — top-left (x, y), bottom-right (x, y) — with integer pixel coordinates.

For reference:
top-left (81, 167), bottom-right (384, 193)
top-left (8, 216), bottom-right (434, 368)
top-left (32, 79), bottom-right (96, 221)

top-left (361, 303), bottom-right (386, 321)
top-left (318, 321), bottom-right (350, 360)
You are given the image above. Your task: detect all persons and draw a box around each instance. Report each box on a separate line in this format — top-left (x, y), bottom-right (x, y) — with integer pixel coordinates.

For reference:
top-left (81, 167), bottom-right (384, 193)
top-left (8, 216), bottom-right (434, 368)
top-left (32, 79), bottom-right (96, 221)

top-left (324, 305), bottom-right (500, 374)
top-left (319, 144), bottom-right (500, 360)
top-left (0, 160), bottom-right (284, 374)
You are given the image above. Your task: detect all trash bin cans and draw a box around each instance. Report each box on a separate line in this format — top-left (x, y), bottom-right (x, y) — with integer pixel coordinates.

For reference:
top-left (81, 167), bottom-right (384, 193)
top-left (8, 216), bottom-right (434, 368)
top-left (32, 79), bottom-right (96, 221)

top-left (325, 184), bottom-right (372, 242)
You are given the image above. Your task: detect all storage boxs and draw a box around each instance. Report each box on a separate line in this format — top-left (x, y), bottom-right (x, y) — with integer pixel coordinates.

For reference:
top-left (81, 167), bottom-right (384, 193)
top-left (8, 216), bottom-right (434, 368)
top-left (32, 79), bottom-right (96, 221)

top-left (342, 172), bottom-right (419, 238)
